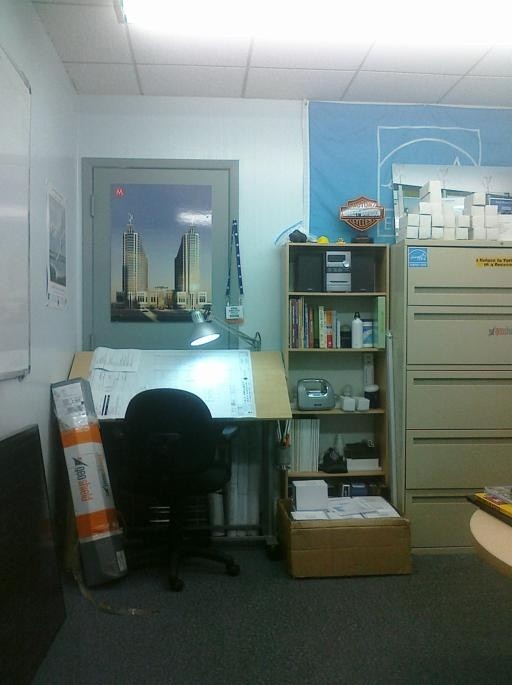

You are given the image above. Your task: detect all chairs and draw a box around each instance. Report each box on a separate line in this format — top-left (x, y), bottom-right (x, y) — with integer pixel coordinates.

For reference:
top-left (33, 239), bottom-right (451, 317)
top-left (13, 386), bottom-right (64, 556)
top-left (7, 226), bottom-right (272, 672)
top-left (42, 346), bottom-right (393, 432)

top-left (101, 388), bottom-right (241, 592)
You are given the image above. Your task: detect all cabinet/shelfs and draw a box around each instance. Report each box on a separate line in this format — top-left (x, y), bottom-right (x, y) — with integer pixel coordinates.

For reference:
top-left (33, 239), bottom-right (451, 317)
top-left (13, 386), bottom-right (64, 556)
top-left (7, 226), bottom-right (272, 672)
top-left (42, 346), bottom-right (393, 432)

top-left (280, 239), bottom-right (512, 555)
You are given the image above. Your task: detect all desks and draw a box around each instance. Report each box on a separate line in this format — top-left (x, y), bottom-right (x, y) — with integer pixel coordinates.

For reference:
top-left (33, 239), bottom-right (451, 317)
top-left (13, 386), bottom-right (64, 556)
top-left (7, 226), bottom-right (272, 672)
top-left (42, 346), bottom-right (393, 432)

top-left (57, 345), bottom-right (293, 560)
top-left (468, 507), bottom-right (511, 582)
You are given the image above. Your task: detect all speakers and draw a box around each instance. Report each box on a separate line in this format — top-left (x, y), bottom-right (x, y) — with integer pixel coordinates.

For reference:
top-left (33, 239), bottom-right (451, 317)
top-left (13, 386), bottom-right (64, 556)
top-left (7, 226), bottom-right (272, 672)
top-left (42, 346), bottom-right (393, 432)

top-left (325, 250), bottom-right (351, 293)
top-left (294, 254), bottom-right (323, 292)
top-left (352, 255), bottom-right (377, 292)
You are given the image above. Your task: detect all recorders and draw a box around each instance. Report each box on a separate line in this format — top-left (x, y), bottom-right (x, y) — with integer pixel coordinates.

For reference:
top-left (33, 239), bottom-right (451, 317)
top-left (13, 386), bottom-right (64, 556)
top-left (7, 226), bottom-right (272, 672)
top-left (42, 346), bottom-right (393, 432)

top-left (296, 378), bottom-right (336, 411)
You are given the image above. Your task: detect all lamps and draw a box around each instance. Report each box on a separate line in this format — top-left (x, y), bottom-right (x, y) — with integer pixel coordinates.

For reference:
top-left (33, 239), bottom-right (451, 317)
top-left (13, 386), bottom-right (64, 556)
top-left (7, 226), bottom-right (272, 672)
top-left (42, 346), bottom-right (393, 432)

top-left (188, 305), bottom-right (262, 351)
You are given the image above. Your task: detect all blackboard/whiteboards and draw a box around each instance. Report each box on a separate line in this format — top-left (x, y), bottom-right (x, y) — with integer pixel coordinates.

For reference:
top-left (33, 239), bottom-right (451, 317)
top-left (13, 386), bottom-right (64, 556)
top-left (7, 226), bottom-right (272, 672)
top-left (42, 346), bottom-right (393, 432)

top-left (0, 46), bottom-right (31, 380)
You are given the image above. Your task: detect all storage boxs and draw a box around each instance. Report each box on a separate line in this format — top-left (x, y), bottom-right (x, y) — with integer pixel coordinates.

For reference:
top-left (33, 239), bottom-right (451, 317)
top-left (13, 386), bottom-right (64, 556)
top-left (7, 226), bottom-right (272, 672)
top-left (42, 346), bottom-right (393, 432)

top-left (50, 376), bottom-right (128, 589)
top-left (277, 498), bottom-right (414, 579)
top-left (394, 178), bottom-right (510, 240)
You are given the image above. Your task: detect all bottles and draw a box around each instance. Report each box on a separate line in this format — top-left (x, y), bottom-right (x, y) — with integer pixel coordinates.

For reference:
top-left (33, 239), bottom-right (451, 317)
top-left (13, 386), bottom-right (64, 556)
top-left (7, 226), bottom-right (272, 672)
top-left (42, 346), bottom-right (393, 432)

top-left (351, 311), bottom-right (364, 349)
top-left (363, 384), bottom-right (381, 409)
top-left (334, 434), bottom-right (345, 456)
top-left (339, 324), bottom-right (351, 349)
top-left (360, 319), bottom-right (373, 348)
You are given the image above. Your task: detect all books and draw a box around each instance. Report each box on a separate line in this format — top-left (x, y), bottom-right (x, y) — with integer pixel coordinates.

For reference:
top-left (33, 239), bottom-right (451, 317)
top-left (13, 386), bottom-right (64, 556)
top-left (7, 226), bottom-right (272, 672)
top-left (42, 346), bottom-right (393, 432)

top-left (288, 418), bottom-right (320, 472)
top-left (474, 492), bottom-right (511, 519)
top-left (485, 485), bottom-right (511, 505)
top-left (287, 296), bottom-right (342, 350)
top-left (464, 494), bottom-right (511, 527)
